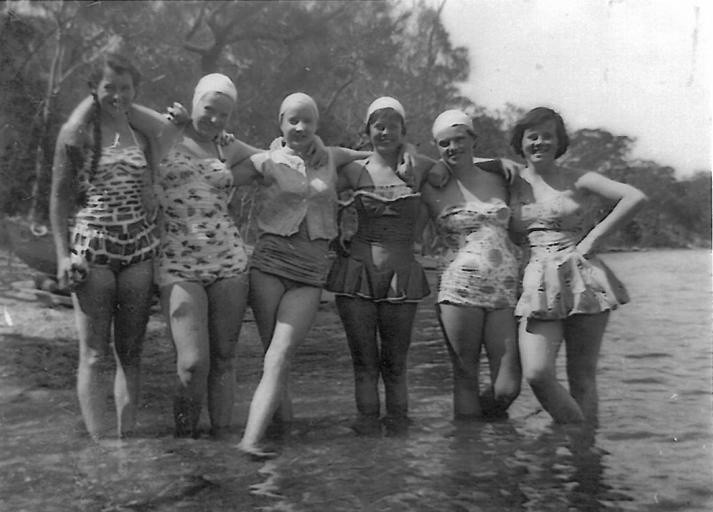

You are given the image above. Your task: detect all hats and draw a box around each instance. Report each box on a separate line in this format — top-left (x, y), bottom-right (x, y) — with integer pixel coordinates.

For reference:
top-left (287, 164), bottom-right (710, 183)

top-left (278, 91), bottom-right (320, 121)
top-left (365, 95), bottom-right (406, 128)
top-left (431, 108), bottom-right (474, 138)
top-left (190, 71), bottom-right (239, 107)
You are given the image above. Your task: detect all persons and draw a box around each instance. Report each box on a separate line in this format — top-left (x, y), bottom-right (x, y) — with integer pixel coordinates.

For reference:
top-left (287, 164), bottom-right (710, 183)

top-left (266, 95), bottom-right (518, 423)
top-left (425, 105), bottom-right (649, 427)
top-left (60, 69), bottom-right (331, 445)
top-left (324, 110), bottom-right (522, 423)
top-left (46, 54), bottom-right (237, 446)
top-left (156, 90), bottom-right (420, 457)
